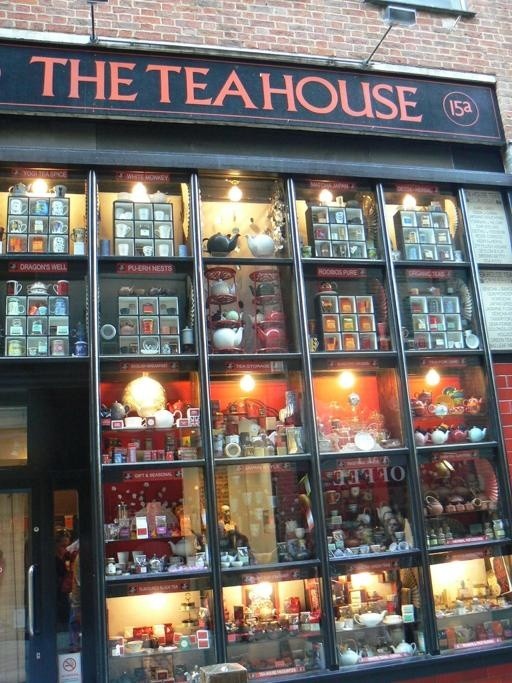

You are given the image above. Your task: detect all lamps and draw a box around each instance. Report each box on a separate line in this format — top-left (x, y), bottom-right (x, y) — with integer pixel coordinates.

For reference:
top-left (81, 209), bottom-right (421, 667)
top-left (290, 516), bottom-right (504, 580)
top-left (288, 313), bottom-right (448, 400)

top-left (362, 6), bottom-right (417, 68)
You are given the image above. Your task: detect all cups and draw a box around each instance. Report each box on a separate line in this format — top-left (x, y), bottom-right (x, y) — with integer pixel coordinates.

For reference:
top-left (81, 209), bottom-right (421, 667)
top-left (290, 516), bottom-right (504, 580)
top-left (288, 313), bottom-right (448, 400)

top-left (10, 219), bottom-right (28, 233)
top-left (115, 224), bottom-right (131, 238)
top-left (50, 340), bottom-right (66, 357)
top-left (335, 212), bottom-right (344, 224)
top-left (143, 246), bottom-right (154, 257)
top-left (48, 184), bottom-right (67, 197)
top-left (136, 554), bottom-right (146, 567)
top-left (118, 244), bottom-right (129, 256)
top-left (301, 245), bottom-right (311, 259)
top-left (53, 237), bottom-right (65, 253)
top-left (137, 207), bottom-right (149, 221)
top-left (120, 309), bottom-right (130, 315)
top-left (7, 340), bottom-right (26, 356)
top-left (367, 248), bottom-right (376, 259)
top-left (10, 200), bottom-right (28, 214)
top-left (117, 551), bottom-right (130, 563)
top-left (155, 211), bottom-right (163, 221)
top-left (7, 280), bottom-right (23, 296)
top-left (70, 227), bottom-right (86, 242)
top-left (155, 225), bottom-right (170, 239)
top-left (167, 308), bottom-right (177, 315)
top-left (158, 244), bottom-right (169, 257)
top-left (131, 550), bottom-right (144, 562)
top-left (10, 237), bottom-right (23, 253)
top-left (51, 201), bottom-right (68, 216)
top-left (52, 280), bottom-right (70, 296)
top-left (142, 319), bottom-right (154, 334)
top-left (50, 220), bottom-right (68, 235)
top-left (123, 417), bottom-right (147, 429)
top-left (116, 207), bottom-right (124, 221)
top-left (232, 562), bottom-right (243, 568)
top-left (50, 299), bottom-right (66, 316)
top-left (384, 614), bottom-right (401, 622)
top-left (8, 302), bottom-right (25, 315)
top-left (221, 563), bottom-right (230, 568)
top-left (335, 620), bottom-right (345, 630)
top-left (74, 340), bottom-right (88, 357)
top-left (182, 325), bottom-right (194, 345)
top-left (326, 490), bottom-right (340, 506)
top-left (30, 200), bottom-right (49, 215)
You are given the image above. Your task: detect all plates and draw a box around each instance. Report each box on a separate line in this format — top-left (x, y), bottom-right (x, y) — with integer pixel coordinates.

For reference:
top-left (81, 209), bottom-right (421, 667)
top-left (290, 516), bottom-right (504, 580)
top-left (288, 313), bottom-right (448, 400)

top-left (141, 349), bottom-right (159, 354)
top-left (383, 619), bottom-right (402, 623)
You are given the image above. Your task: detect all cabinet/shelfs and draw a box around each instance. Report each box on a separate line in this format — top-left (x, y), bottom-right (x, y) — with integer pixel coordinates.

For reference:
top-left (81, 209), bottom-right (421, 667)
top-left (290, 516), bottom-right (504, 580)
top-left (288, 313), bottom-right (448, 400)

top-left (1, 155), bottom-right (512, 681)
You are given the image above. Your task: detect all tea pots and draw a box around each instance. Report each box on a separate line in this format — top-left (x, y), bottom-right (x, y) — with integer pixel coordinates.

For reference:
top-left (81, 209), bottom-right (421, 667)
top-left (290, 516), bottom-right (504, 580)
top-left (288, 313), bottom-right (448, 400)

top-left (167, 537), bottom-right (194, 557)
top-left (428, 403), bottom-right (448, 415)
top-left (245, 232), bottom-right (274, 256)
top-left (211, 310), bottom-right (225, 321)
top-left (465, 426), bottom-right (488, 442)
top-left (339, 648), bottom-right (363, 666)
top-left (265, 309), bottom-right (286, 321)
top-left (426, 496), bottom-right (444, 516)
top-left (71, 320), bottom-right (86, 340)
top-left (415, 430), bottom-right (428, 446)
top-left (411, 400), bottom-right (428, 416)
top-left (146, 406), bottom-right (183, 429)
top-left (212, 327), bottom-right (244, 350)
top-left (120, 321), bottom-right (136, 335)
top-left (415, 389), bottom-right (433, 404)
top-left (149, 288), bottom-right (162, 296)
top-left (463, 396), bottom-right (482, 415)
top-left (8, 181), bottom-right (33, 196)
top-left (201, 231), bottom-right (240, 258)
top-left (205, 279), bottom-right (238, 302)
top-left (389, 640), bottom-right (417, 656)
top-left (118, 285), bottom-right (135, 297)
top-left (223, 307), bottom-right (245, 321)
top-left (102, 401), bottom-right (131, 420)
top-left (248, 312), bottom-right (265, 324)
top-left (220, 551), bottom-right (238, 562)
top-left (255, 327), bottom-right (291, 352)
top-left (249, 284), bottom-right (276, 297)
top-left (353, 610), bottom-right (387, 626)
top-left (146, 552), bottom-right (167, 572)
top-left (426, 429), bottom-right (450, 445)
top-left (27, 280), bottom-right (52, 295)
top-left (449, 427), bottom-right (468, 442)
